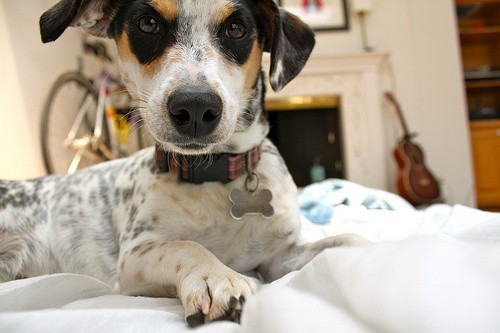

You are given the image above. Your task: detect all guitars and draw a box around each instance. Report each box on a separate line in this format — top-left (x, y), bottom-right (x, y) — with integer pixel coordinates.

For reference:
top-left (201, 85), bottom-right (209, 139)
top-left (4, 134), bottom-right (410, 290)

top-left (384, 90), bottom-right (443, 206)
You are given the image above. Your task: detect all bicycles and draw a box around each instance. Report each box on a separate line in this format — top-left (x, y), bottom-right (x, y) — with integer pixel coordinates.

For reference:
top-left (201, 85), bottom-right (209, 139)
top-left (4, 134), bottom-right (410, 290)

top-left (41, 37), bottom-right (147, 176)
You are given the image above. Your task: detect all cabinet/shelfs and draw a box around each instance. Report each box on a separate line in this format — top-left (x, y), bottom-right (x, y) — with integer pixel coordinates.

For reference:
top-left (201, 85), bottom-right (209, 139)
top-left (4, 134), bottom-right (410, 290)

top-left (453, 0), bottom-right (500, 120)
top-left (467, 121), bottom-right (500, 213)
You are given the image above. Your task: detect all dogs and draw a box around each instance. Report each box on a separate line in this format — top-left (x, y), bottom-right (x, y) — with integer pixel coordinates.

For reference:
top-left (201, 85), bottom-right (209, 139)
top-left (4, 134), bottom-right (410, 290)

top-left (0, 0), bottom-right (371, 331)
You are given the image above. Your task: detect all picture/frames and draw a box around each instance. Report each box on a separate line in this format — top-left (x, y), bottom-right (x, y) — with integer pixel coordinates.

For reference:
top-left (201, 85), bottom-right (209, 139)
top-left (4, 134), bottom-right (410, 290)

top-left (277, 0), bottom-right (348, 33)
